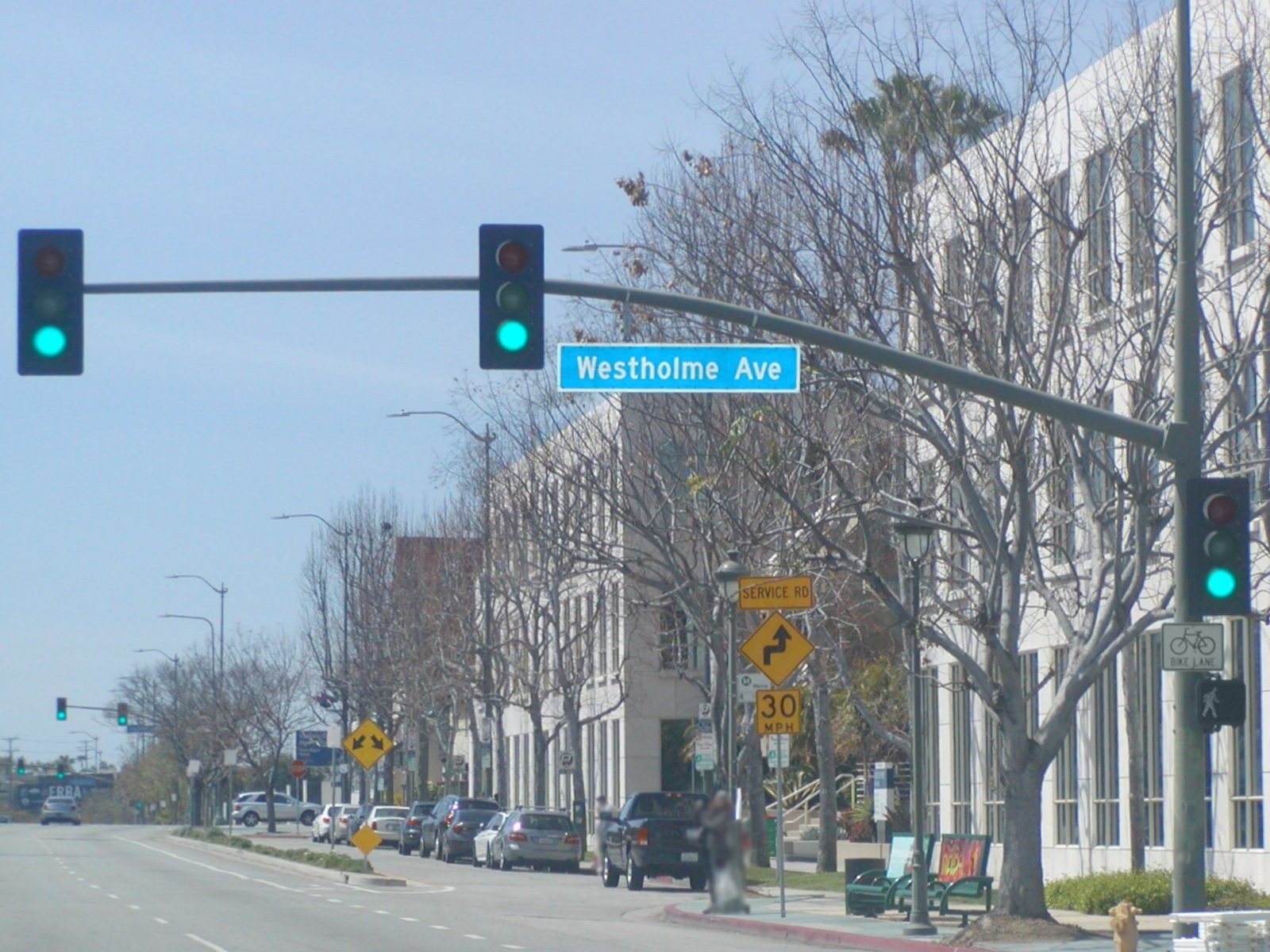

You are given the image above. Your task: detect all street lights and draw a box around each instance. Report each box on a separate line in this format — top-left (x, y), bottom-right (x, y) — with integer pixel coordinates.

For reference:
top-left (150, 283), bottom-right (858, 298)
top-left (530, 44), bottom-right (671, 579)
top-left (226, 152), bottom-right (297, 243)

top-left (713, 551), bottom-right (749, 916)
top-left (892, 493), bottom-right (940, 935)
top-left (386, 410), bottom-right (494, 797)
top-left (70, 730), bottom-right (99, 768)
top-left (122, 572), bottom-right (226, 778)
top-left (271, 512), bottom-right (355, 804)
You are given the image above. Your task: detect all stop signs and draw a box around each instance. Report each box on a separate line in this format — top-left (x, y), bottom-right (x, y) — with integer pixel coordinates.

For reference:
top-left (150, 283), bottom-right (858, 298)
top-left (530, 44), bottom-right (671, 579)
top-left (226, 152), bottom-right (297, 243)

top-left (289, 758), bottom-right (308, 779)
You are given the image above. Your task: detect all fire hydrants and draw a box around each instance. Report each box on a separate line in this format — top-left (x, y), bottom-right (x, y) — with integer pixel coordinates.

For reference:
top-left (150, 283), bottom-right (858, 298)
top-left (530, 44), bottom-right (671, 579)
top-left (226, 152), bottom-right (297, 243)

top-left (1109, 899), bottom-right (1143, 952)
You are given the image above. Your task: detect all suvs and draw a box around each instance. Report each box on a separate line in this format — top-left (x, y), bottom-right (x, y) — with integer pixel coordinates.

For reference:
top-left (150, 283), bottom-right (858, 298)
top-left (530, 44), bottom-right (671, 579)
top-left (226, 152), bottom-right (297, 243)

top-left (418, 795), bottom-right (503, 859)
top-left (232, 789), bottom-right (321, 827)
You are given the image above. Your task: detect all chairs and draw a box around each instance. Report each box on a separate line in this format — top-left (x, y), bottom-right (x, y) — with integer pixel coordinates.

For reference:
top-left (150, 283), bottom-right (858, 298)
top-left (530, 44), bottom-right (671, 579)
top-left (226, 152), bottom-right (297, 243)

top-left (846, 832), bottom-right (937, 919)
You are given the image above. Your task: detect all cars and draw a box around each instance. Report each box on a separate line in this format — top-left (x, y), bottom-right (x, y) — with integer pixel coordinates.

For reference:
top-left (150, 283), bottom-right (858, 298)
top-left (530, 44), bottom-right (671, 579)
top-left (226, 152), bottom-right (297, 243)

top-left (333, 804), bottom-right (359, 844)
top-left (311, 803), bottom-right (342, 842)
top-left (360, 805), bottom-right (410, 844)
top-left (492, 807), bottom-right (583, 870)
top-left (398, 800), bottom-right (436, 855)
top-left (470, 813), bottom-right (515, 872)
top-left (444, 809), bottom-right (498, 864)
top-left (41, 795), bottom-right (83, 826)
top-left (346, 805), bottom-right (373, 844)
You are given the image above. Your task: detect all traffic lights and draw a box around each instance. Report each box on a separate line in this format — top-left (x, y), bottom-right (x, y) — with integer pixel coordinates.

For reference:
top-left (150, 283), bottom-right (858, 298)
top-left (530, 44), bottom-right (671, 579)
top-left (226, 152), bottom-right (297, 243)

top-left (1187, 475), bottom-right (1252, 617)
top-left (115, 703), bottom-right (128, 727)
top-left (16, 758), bottom-right (26, 775)
top-left (55, 764), bottom-right (64, 779)
top-left (477, 224), bottom-right (545, 370)
top-left (56, 696), bottom-right (67, 719)
top-left (16, 228), bottom-right (84, 375)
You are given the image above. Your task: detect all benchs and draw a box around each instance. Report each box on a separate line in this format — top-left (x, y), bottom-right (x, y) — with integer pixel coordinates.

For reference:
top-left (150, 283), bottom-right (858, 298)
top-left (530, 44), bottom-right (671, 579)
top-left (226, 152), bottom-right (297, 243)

top-left (895, 833), bottom-right (995, 929)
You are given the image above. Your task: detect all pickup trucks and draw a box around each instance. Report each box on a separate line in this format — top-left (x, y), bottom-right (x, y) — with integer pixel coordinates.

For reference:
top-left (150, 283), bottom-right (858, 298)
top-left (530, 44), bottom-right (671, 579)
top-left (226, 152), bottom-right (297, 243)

top-left (599, 791), bottom-right (711, 890)
